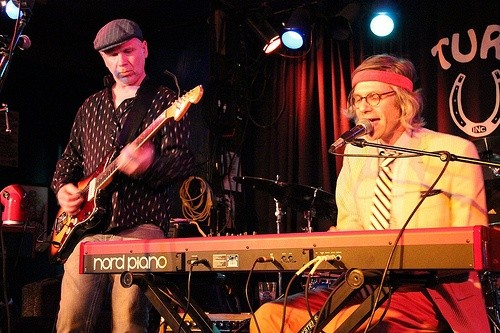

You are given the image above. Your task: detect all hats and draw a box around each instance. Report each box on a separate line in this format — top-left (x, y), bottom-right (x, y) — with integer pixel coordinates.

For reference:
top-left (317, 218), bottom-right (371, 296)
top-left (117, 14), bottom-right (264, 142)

top-left (94, 18), bottom-right (141, 52)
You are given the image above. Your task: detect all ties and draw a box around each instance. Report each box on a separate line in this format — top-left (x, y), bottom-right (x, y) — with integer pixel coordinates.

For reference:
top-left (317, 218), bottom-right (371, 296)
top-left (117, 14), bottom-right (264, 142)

top-left (355, 152), bottom-right (392, 300)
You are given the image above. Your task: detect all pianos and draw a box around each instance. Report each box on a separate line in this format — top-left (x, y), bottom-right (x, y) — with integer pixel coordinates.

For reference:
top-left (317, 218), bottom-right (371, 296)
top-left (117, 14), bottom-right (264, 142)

top-left (78, 224), bottom-right (500, 276)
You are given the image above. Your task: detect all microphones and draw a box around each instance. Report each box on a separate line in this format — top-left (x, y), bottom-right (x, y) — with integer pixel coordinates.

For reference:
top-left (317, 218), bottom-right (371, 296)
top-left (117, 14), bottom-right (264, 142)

top-left (0, 35), bottom-right (32, 50)
top-left (330, 118), bottom-right (373, 154)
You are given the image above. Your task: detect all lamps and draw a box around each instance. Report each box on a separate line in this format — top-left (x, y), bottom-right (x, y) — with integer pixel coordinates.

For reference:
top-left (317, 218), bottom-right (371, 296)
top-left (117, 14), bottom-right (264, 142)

top-left (281, 7), bottom-right (312, 50)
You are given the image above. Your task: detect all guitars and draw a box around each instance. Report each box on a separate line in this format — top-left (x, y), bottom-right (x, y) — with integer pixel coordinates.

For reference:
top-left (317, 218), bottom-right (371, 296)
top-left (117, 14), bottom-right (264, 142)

top-left (49, 84), bottom-right (204, 263)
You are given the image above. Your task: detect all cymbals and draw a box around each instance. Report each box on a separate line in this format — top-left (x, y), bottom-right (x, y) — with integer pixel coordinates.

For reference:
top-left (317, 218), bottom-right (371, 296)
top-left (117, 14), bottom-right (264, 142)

top-left (233, 175), bottom-right (288, 196)
top-left (282, 181), bottom-right (334, 202)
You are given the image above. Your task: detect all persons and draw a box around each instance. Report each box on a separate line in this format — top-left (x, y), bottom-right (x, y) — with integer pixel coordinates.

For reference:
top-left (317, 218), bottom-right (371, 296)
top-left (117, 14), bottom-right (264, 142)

top-left (249, 54), bottom-right (491, 333)
top-left (50, 18), bottom-right (197, 333)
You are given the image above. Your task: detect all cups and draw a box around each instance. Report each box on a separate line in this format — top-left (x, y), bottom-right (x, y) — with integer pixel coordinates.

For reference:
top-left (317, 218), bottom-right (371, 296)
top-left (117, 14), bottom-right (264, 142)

top-left (258, 282), bottom-right (276, 304)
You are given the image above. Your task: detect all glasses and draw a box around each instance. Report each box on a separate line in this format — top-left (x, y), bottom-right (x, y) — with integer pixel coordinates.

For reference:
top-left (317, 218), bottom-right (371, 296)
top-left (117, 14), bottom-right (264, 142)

top-left (349, 91), bottom-right (395, 109)
